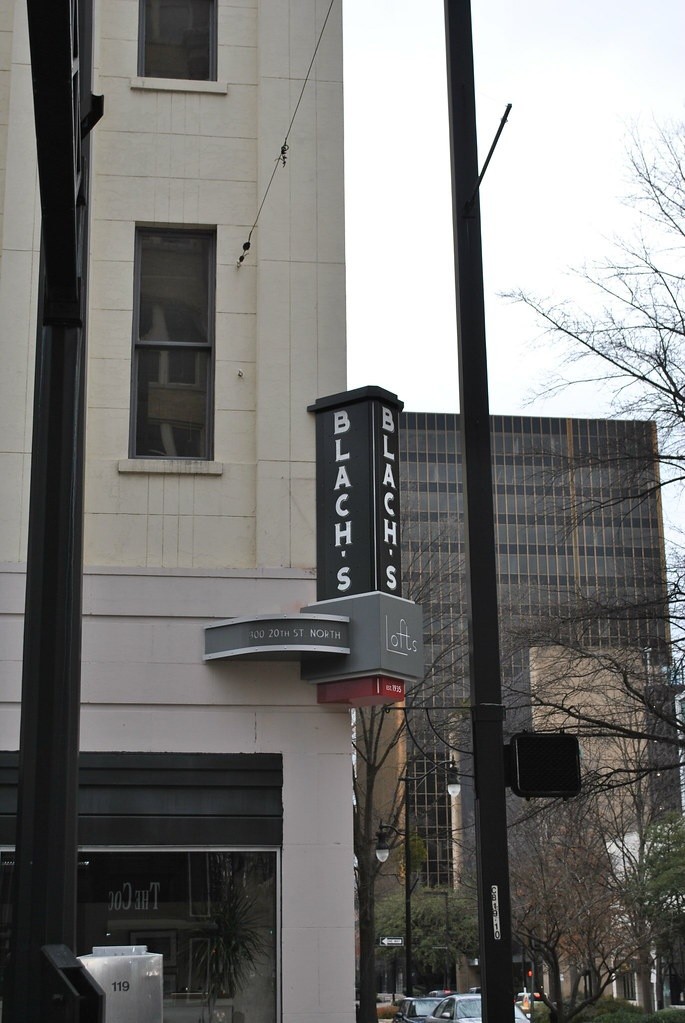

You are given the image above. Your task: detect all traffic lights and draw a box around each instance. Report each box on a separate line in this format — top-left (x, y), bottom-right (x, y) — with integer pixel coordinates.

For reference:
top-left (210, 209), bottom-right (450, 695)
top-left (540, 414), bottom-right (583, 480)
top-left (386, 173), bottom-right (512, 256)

top-left (528, 969), bottom-right (532, 977)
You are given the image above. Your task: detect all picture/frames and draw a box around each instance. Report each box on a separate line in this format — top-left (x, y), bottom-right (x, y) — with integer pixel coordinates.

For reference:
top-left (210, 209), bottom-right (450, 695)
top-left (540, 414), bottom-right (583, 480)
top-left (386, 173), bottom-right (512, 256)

top-left (129, 928), bottom-right (178, 967)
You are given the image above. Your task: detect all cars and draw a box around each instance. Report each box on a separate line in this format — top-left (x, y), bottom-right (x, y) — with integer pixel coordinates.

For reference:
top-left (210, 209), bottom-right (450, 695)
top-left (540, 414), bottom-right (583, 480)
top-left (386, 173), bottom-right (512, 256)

top-left (392, 998), bottom-right (445, 1023)
top-left (424, 993), bottom-right (531, 1023)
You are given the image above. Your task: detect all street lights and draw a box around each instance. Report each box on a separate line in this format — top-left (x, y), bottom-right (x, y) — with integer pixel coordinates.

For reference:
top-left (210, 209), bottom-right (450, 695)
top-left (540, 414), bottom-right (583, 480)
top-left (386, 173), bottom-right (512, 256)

top-left (375, 752), bottom-right (460, 998)
